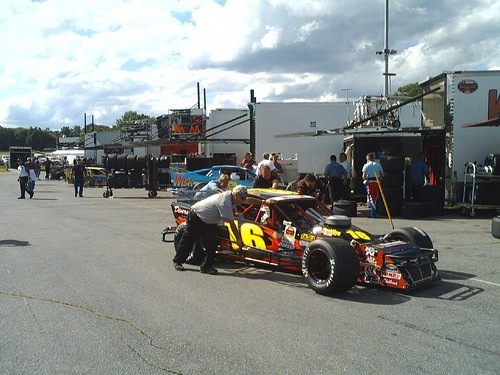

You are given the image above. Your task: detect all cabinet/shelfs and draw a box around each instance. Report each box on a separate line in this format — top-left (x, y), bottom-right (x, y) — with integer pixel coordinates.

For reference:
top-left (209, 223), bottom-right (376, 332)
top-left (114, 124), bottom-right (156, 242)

top-left (462, 162), bottom-right (500, 216)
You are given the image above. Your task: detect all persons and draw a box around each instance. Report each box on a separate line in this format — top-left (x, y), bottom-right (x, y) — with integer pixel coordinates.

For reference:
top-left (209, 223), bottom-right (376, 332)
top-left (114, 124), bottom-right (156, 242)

top-left (297, 172), bottom-right (329, 204)
top-left (254, 151), bottom-right (276, 177)
top-left (42, 157), bottom-right (54, 180)
top-left (60, 155), bottom-right (97, 168)
top-left (408, 149), bottom-right (429, 203)
top-left (323, 154), bottom-right (348, 201)
top-left (15, 158), bottom-right (34, 199)
top-left (72, 160), bottom-right (87, 198)
top-left (362, 152), bottom-right (385, 219)
top-left (33, 157), bottom-right (40, 181)
top-left (271, 156), bottom-right (284, 175)
top-left (24, 156), bottom-right (36, 190)
top-left (191, 174), bottom-right (233, 206)
top-left (238, 150), bottom-right (257, 170)
top-left (252, 165), bottom-right (279, 189)
top-left (173, 183), bottom-right (248, 275)
top-left (338, 152), bottom-right (353, 200)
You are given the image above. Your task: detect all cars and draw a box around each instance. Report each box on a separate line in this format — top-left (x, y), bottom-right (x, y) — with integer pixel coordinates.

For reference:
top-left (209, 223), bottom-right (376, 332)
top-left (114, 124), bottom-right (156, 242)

top-left (33, 154), bottom-right (108, 188)
top-left (2, 154), bottom-right (10, 164)
top-left (0, 156), bottom-right (4, 166)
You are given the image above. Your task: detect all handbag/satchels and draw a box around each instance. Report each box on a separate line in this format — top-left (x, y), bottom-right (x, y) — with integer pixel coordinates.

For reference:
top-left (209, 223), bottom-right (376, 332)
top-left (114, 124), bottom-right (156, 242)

top-left (27, 180), bottom-right (33, 191)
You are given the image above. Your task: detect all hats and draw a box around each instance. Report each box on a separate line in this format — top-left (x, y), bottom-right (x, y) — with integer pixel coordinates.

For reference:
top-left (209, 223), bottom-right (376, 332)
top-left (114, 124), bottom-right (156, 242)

top-left (233, 185), bottom-right (251, 197)
top-left (219, 174), bottom-right (231, 181)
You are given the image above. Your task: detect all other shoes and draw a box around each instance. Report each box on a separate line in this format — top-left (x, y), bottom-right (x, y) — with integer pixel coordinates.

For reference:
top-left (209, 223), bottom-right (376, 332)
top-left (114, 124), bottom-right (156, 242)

top-left (75, 193), bottom-right (77, 197)
top-left (80, 195), bottom-right (83, 197)
top-left (200, 266), bottom-right (218, 275)
top-left (18, 197), bottom-right (25, 199)
top-left (30, 192), bottom-right (34, 199)
top-left (174, 261), bottom-right (184, 271)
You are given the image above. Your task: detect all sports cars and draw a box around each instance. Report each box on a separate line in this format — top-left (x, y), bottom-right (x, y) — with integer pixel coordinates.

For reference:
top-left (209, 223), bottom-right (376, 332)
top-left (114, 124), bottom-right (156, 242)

top-left (170, 165), bottom-right (258, 196)
top-left (160, 188), bottom-right (440, 296)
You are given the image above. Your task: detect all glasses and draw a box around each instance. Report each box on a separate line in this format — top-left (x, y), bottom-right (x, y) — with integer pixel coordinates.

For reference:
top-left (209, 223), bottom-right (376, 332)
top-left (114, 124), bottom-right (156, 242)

top-left (240, 196), bottom-right (246, 201)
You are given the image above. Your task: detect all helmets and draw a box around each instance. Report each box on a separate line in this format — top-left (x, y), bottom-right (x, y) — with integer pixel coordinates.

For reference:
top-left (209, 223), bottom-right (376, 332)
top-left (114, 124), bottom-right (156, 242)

top-left (260, 205), bottom-right (278, 225)
top-left (230, 172), bottom-right (240, 180)
